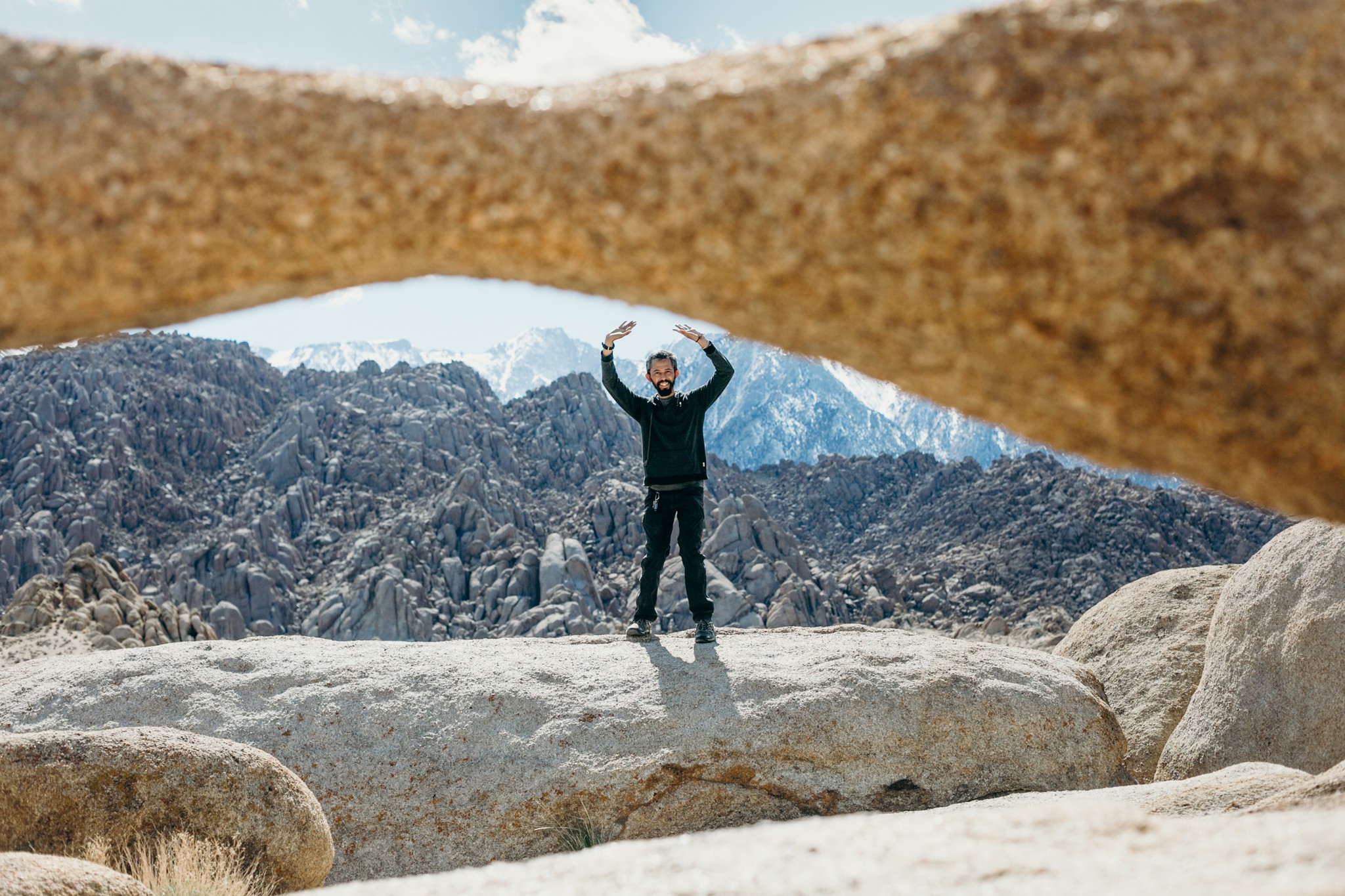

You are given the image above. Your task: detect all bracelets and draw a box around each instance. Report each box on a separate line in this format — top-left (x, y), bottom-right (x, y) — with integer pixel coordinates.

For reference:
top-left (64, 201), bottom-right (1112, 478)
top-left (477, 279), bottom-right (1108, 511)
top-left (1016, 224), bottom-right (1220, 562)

top-left (696, 333), bottom-right (703, 342)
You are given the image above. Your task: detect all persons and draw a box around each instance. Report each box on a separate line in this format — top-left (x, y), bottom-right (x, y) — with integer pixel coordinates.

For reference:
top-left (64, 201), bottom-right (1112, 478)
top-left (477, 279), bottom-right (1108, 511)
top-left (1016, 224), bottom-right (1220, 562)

top-left (601, 321), bottom-right (735, 644)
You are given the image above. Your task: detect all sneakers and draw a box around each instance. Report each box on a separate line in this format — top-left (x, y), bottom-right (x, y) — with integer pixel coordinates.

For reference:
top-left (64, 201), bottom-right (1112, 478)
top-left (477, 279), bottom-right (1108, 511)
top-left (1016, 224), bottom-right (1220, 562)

top-left (626, 618), bottom-right (652, 636)
top-left (695, 618), bottom-right (716, 643)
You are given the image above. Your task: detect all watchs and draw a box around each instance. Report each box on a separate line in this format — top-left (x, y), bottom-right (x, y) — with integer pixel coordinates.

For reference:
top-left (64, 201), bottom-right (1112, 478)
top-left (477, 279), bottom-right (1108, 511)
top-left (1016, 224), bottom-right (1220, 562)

top-left (602, 342), bottom-right (615, 350)
